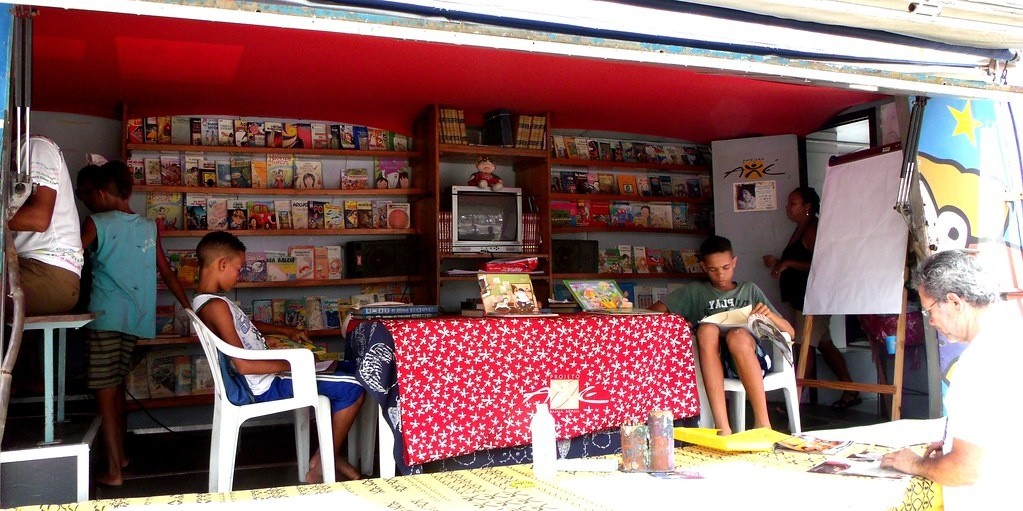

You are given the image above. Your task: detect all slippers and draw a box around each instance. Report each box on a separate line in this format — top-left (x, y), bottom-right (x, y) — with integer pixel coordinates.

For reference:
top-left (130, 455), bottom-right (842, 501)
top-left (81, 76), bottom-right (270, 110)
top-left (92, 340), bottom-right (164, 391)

top-left (95, 481), bottom-right (128, 490)
top-left (121, 458), bottom-right (132, 474)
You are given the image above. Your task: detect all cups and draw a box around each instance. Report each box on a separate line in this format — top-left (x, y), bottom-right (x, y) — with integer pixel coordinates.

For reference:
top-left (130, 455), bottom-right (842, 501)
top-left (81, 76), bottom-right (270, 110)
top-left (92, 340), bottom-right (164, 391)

top-left (885, 335), bottom-right (897, 355)
top-left (619, 426), bottom-right (647, 471)
top-left (649, 410), bottom-right (675, 472)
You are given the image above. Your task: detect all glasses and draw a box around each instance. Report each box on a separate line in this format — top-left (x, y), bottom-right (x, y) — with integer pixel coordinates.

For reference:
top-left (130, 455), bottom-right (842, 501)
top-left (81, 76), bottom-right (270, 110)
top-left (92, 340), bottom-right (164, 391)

top-left (922, 299), bottom-right (941, 317)
top-left (75, 188), bottom-right (93, 197)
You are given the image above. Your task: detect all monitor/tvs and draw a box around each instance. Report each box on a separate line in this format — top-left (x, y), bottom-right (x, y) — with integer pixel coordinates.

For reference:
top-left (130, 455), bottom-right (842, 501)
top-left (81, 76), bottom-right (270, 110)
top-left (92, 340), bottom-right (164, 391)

top-left (446, 186), bottom-right (522, 252)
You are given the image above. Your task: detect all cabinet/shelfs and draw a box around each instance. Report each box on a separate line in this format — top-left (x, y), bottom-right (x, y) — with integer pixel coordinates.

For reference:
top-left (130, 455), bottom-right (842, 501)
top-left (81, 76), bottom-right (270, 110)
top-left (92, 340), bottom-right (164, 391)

top-left (121, 147), bottom-right (424, 437)
top-left (414, 103), bottom-right (553, 309)
top-left (552, 156), bottom-right (713, 308)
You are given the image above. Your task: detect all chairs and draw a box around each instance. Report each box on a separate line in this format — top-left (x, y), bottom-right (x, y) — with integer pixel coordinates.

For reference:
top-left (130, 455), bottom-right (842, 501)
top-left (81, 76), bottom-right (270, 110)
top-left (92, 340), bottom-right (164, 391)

top-left (685, 330), bottom-right (803, 437)
top-left (184, 312), bottom-right (339, 492)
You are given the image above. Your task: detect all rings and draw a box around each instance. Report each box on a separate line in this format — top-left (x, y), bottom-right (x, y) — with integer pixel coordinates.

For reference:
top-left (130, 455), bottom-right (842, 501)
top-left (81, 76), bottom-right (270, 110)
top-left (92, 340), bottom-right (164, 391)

top-left (775, 271), bottom-right (778, 273)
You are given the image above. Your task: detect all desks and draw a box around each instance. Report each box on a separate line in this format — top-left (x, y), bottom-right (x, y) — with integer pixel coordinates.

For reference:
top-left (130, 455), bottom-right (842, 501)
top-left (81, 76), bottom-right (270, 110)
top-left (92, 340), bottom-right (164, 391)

top-left (342, 310), bottom-right (700, 488)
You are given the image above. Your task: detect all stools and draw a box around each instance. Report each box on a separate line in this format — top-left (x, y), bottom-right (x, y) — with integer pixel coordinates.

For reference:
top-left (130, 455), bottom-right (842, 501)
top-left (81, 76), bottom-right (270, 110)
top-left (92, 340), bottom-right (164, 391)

top-left (13, 310), bottom-right (106, 448)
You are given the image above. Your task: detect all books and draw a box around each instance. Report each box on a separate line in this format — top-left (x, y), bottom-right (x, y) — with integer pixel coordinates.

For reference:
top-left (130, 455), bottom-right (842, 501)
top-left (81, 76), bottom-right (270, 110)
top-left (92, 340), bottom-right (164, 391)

top-left (522, 213), bottom-right (540, 254)
top-left (438, 211), bottom-right (452, 254)
top-left (124, 117), bottom-right (413, 230)
top-left (446, 273), bottom-right (686, 317)
top-left (771, 433), bottom-right (930, 481)
top-left (698, 303), bottom-right (793, 366)
top-left (124, 245), bottom-right (439, 400)
top-left (549, 136), bottom-right (712, 229)
top-left (598, 245), bottom-right (706, 273)
top-left (439, 108), bottom-right (547, 150)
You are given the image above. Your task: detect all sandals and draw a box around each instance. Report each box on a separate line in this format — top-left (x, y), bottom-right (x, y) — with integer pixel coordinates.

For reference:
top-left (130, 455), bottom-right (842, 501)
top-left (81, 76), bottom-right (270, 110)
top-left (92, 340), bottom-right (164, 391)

top-left (831, 391), bottom-right (862, 410)
top-left (776, 406), bottom-right (787, 415)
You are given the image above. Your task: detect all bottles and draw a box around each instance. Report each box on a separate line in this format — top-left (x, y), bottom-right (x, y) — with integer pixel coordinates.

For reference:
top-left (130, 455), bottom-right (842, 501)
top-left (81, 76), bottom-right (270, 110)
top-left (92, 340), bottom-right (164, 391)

top-left (530, 403), bottom-right (557, 480)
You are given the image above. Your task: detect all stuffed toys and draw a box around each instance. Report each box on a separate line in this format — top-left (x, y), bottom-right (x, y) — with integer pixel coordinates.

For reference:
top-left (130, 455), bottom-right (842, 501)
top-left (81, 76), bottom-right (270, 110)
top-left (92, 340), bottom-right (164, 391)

top-left (468, 155), bottom-right (504, 192)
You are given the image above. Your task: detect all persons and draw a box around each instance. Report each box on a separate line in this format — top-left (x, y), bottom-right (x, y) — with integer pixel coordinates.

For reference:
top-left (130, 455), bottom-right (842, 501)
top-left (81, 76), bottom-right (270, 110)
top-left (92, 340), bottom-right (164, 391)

top-left (82, 160), bottom-right (193, 485)
top-left (4, 85), bottom-right (84, 394)
top-left (879, 249), bottom-right (1023, 511)
top-left (486, 225), bottom-right (496, 240)
top-left (191, 231), bottom-right (366, 484)
top-left (737, 184), bottom-right (755, 208)
top-left (647, 235), bottom-right (795, 440)
top-left (74, 165), bottom-right (103, 213)
top-left (762, 186), bottom-right (863, 412)
top-left (470, 221), bottom-right (478, 233)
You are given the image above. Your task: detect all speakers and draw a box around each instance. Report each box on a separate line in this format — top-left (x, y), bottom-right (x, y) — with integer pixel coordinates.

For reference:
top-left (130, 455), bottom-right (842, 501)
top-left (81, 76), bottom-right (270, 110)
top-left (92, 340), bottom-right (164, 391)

top-left (551, 239), bottom-right (599, 273)
top-left (345, 237), bottom-right (420, 277)
